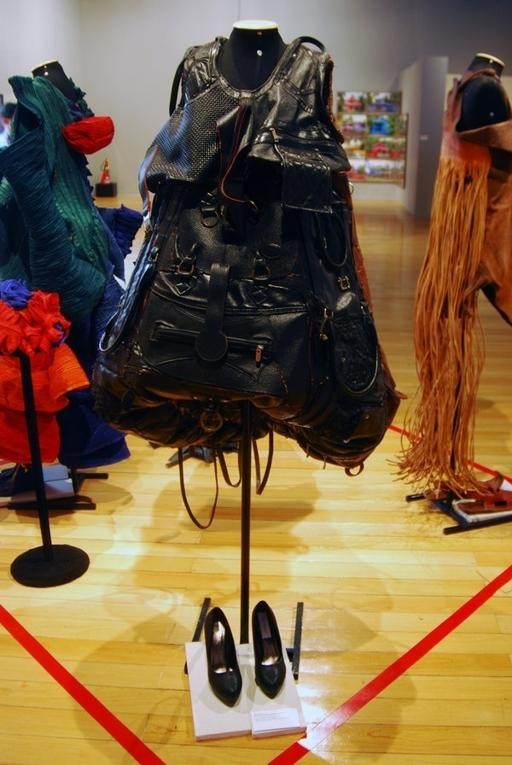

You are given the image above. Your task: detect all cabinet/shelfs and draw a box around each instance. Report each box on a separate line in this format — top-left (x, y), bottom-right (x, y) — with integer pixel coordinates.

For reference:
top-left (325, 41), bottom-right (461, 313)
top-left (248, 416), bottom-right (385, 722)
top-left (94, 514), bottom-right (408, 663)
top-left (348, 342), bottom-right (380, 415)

top-left (334, 109), bottom-right (411, 190)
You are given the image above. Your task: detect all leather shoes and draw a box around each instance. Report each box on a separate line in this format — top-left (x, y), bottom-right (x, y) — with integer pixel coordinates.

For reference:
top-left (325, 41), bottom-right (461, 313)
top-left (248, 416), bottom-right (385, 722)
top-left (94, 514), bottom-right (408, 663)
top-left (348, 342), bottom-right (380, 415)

top-left (252, 600), bottom-right (286, 698)
top-left (204, 607), bottom-right (243, 707)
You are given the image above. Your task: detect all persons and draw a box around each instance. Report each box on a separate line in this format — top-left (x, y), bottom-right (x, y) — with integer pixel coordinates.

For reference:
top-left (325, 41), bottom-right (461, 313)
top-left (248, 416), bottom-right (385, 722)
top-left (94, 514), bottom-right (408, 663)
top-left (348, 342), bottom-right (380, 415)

top-left (393, 39), bottom-right (511, 502)
top-left (82, 13), bottom-right (401, 473)
top-left (1, 50), bottom-right (131, 476)
